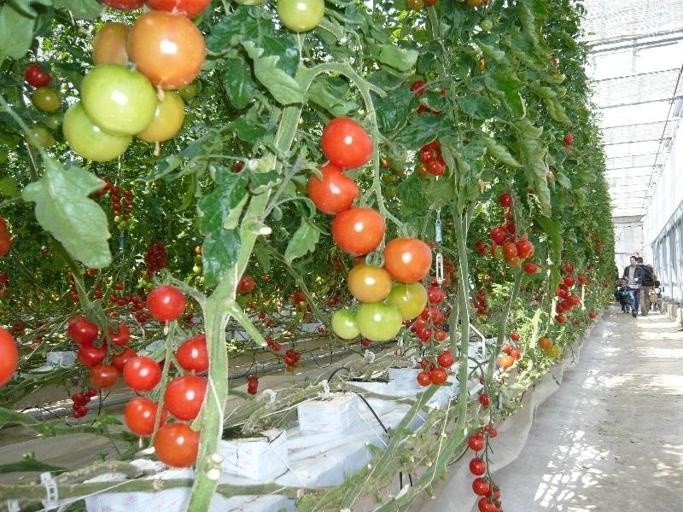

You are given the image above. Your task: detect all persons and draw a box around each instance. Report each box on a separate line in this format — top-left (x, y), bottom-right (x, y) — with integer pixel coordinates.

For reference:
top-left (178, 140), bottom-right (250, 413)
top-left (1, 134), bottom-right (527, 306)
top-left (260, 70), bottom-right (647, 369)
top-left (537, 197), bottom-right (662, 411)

top-left (616, 255), bottom-right (661, 319)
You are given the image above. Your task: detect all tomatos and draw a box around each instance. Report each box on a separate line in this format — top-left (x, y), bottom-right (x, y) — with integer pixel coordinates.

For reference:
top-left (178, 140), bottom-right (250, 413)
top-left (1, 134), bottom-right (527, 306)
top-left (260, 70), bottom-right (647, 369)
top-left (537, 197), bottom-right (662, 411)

top-left (0, 0), bottom-right (612, 512)
